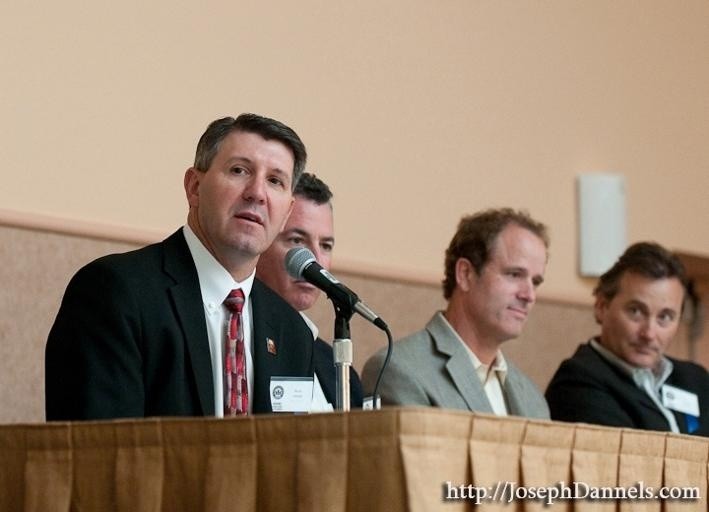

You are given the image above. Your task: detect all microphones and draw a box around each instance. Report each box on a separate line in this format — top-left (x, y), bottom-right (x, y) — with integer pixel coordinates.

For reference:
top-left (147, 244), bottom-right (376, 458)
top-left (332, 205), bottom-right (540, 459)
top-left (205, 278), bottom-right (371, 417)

top-left (284, 246), bottom-right (386, 330)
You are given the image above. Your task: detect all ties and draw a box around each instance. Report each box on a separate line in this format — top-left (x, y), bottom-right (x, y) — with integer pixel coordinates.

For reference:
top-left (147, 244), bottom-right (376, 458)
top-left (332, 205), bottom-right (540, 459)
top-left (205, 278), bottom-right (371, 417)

top-left (225, 289), bottom-right (249, 413)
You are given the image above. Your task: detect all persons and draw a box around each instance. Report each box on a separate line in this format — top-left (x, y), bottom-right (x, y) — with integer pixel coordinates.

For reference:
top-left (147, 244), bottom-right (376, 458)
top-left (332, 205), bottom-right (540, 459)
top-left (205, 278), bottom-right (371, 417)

top-left (356, 209), bottom-right (558, 419)
top-left (254, 172), bottom-right (368, 409)
top-left (541, 238), bottom-right (707, 442)
top-left (42, 116), bottom-right (321, 423)
